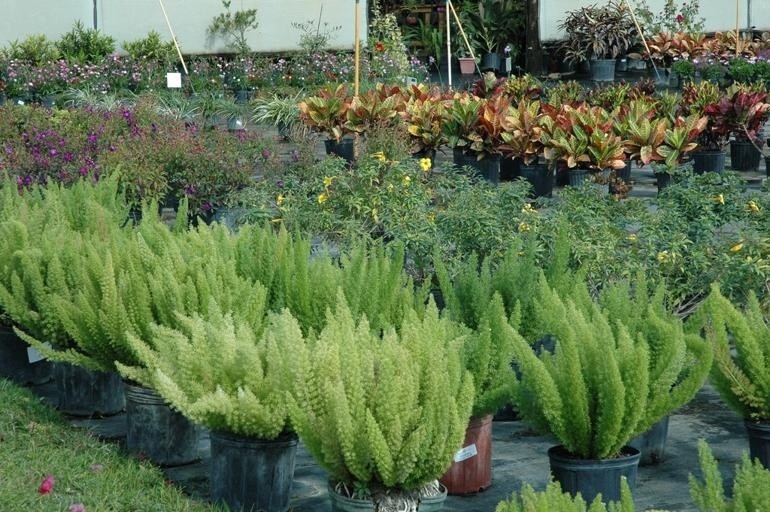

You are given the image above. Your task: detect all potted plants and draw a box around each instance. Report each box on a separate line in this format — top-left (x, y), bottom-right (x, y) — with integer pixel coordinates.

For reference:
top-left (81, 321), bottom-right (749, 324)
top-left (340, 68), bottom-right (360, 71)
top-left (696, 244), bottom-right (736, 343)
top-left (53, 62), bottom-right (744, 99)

top-left (0, 0), bottom-right (770, 511)
